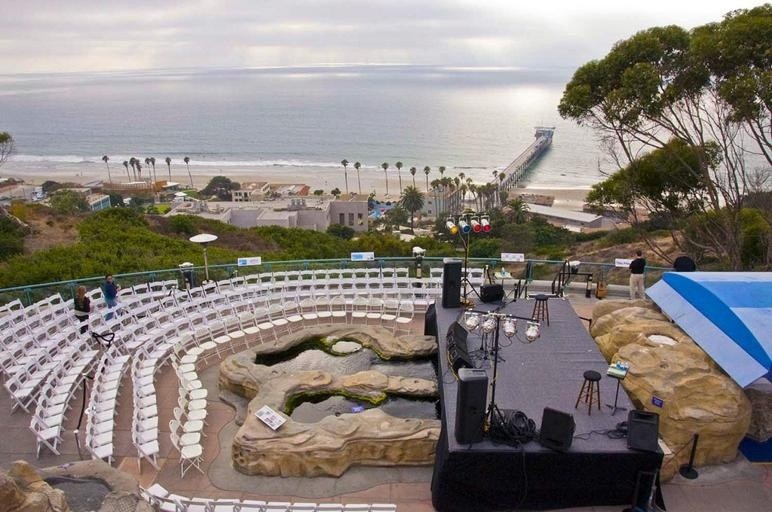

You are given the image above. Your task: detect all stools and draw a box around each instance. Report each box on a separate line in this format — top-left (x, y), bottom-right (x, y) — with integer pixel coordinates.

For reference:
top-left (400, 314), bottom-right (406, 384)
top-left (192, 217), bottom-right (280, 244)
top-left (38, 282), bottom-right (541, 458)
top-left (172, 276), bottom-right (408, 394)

top-left (532, 294), bottom-right (549, 326)
top-left (574, 369), bottom-right (601, 417)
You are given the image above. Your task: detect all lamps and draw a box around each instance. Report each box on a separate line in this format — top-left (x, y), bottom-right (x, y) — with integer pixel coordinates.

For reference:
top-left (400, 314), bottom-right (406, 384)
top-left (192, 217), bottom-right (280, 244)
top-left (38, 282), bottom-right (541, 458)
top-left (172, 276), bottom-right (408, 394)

top-left (442, 213), bottom-right (491, 306)
top-left (463, 308), bottom-right (541, 451)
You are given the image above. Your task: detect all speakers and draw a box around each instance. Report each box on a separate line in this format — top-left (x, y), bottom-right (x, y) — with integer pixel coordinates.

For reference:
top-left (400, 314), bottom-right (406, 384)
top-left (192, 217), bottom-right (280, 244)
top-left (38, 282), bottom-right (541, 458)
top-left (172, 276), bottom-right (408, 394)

top-left (447, 321), bottom-right (467, 343)
top-left (480, 284), bottom-right (503, 302)
top-left (441, 257), bottom-right (462, 308)
top-left (627, 410), bottom-right (659, 452)
top-left (454, 368), bottom-right (488, 444)
top-left (539, 407), bottom-right (575, 453)
top-left (446, 346), bottom-right (473, 373)
top-left (446, 332), bottom-right (467, 353)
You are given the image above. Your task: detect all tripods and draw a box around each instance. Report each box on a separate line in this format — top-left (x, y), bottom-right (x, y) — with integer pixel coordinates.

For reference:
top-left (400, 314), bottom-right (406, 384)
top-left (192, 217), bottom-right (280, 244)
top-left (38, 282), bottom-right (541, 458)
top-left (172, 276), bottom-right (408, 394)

top-left (461, 234), bottom-right (483, 305)
top-left (469, 328), bottom-right (505, 368)
top-left (468, 322), bottom-right (519, 450)
top-left (606, 378), bottom-right (627, 416)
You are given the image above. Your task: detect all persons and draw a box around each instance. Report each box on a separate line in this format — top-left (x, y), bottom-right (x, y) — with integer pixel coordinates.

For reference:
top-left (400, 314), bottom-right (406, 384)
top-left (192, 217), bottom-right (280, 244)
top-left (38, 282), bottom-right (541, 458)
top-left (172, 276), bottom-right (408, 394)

top-left (74, 285), bottom-right (91, 334)
top-left (627, 250), bottom-right (645, 301)
top-left (101, 273), bottom-right (122, 323)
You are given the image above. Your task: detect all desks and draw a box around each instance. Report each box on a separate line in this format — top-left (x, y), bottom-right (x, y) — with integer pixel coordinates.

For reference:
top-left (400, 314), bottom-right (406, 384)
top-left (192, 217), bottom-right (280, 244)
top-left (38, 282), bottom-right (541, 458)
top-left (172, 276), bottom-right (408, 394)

top-left (493, 272), bottom-right (513, 291)
top-left (558, 272), bottom-right (593, 298)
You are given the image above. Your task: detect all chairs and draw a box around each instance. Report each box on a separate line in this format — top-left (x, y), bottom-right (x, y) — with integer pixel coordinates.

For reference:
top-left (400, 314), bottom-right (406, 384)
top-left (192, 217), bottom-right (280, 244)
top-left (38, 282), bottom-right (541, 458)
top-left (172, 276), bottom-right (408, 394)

top-left (203, 264), bottom-right (489, 363)
top-left (137, 481), bottom-right (397, 512)
top-left (1, 280), bottom-right (214, 479)
